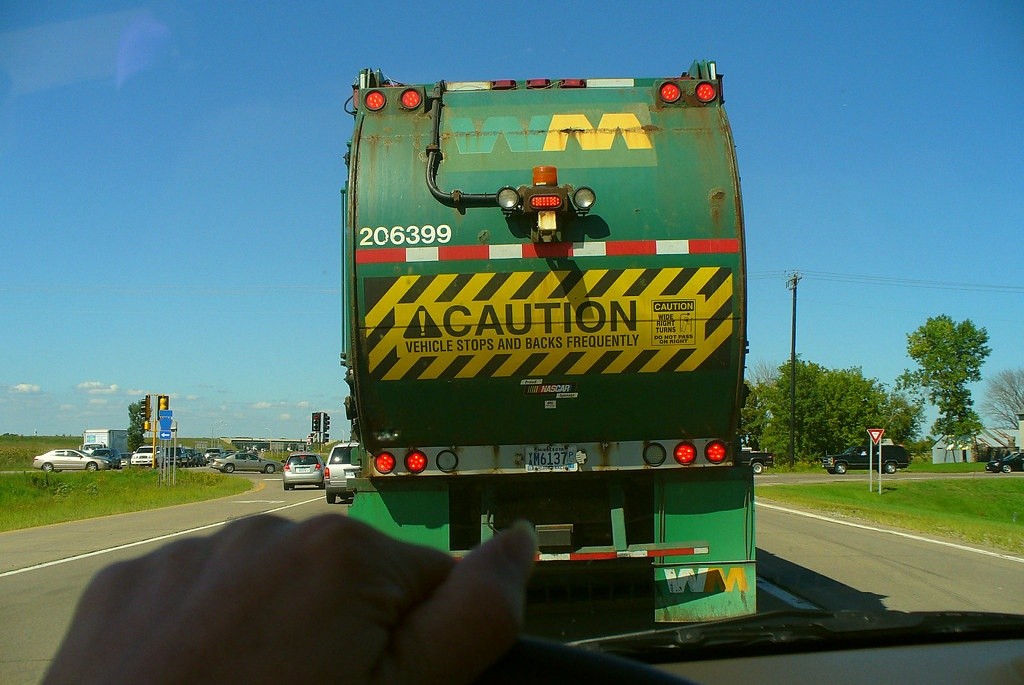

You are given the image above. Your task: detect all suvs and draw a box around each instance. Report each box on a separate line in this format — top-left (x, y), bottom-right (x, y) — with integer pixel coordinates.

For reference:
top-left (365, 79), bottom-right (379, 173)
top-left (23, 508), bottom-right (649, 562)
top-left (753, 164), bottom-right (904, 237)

top-left (203, 448), bottom-right (222, 461)
top-left (324, 442), bottom-right (369, 504)
top-left (130, 446), bottom-right (160, 468)
top-left (91, 448), bottom-right (121, 470)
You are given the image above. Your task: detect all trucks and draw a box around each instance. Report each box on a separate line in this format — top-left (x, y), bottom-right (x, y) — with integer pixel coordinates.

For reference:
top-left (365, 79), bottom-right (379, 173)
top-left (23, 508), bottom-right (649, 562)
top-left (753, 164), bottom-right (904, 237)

top-left (339, 56), bottom-right (758, 632)
top-left (79, 429), bottom-right (130, 453)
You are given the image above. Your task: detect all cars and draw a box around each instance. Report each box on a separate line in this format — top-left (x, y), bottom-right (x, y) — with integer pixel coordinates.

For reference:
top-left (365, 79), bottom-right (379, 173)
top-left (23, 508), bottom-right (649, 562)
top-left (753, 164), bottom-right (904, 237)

top-left (165, 447), bottom-right (207, 468)
top-left (986, 452), bottom-right (1024, 474)
top-left (212, 453), bottom-right (280, 474)
top-left (32, 448), bottom-right (109, 473)
top-left (120, 452), bottom-right (133, 468)
top-left (281, 453), bottom-right (326, 490)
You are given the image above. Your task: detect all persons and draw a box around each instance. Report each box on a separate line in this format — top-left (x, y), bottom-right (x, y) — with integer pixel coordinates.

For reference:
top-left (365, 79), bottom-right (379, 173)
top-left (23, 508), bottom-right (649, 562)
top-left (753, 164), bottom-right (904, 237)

top-left (34, 511), bottom-right (541, 684)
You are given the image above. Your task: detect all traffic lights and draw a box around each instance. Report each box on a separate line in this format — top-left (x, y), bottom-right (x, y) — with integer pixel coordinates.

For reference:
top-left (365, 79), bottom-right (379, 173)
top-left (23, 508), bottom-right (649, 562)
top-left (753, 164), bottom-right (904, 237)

top-left (323, 434), bottom-right (329, 444)
top-left (157, 396), bottom-right (169, 420)
top-left (323, 413), bottom-right (329, 432)
top-left (140, 395), bottom-right (151, 420)
top-left (312, 412), bottom-right (320, 431)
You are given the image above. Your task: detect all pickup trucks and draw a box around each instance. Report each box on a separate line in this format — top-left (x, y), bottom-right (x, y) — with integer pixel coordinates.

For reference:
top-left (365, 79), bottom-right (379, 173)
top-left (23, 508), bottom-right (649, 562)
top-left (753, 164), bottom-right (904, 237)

top-left (822, 444), bottom-right (909, 474)
top-left (741, 448), bottom-right (774, 476)
